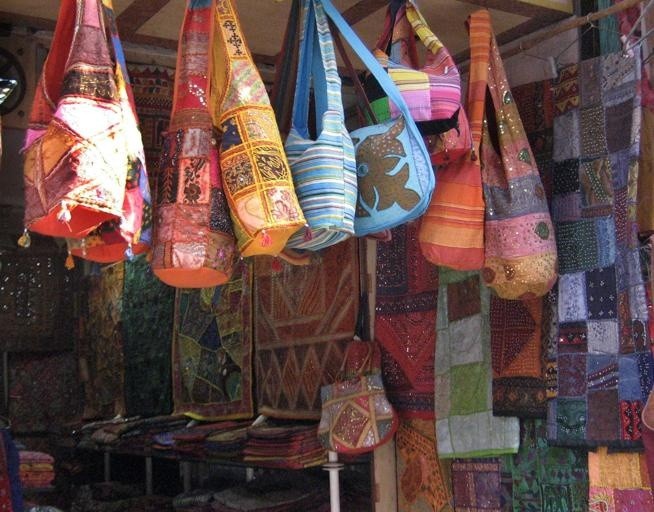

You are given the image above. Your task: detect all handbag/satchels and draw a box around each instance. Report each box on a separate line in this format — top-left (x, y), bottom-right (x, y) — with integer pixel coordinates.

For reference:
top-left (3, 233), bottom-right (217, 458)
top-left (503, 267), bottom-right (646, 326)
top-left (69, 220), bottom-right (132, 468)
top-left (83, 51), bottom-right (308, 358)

top-left (17, 0), bottom-right (152, 270)
top-left (316, 333), bottom-right (400, 458)
top-left (149, 2), bottom-right (558, 300)
